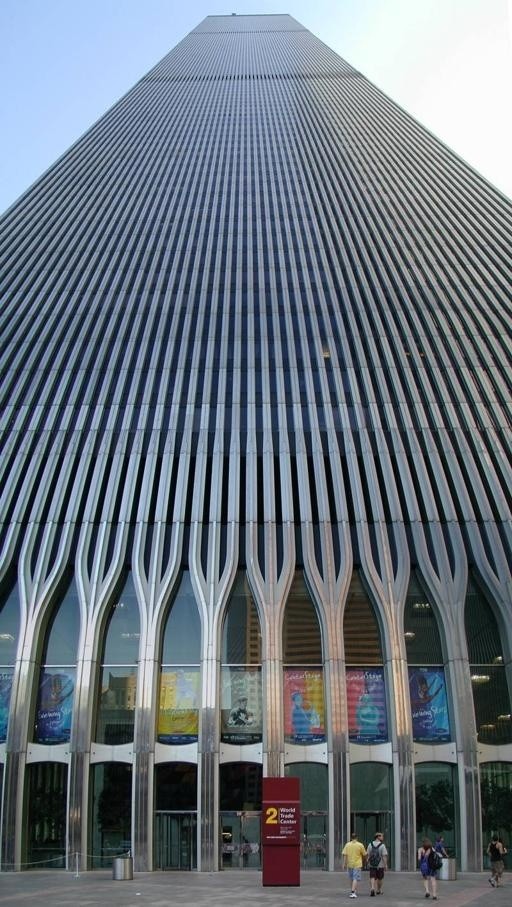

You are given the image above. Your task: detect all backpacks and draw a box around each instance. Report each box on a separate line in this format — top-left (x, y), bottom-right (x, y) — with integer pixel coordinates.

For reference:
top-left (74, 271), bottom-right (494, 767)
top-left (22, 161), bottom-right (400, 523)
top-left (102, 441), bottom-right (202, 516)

top-left (370, 839), bottom-right (384, 866)
top-left (428, 847), bottom-right (442, 869)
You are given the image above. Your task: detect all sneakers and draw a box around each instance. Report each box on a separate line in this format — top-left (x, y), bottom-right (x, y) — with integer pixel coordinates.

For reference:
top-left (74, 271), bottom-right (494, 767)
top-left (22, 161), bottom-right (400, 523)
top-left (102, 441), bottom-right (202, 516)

top-left (370, 890), bottom-right (375, 896)
top-left (426, 893), bottom-right (430, 898)
top-left (349, 893), bottom-right (357, 898)
top-left (377, 890), bottom-right (380, 894)
top-left (489, 879), bottom-right (495, 887)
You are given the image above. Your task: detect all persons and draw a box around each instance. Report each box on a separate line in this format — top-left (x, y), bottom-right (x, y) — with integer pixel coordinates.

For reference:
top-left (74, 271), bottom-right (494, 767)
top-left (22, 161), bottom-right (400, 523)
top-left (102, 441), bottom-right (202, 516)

top-left (291, 692), bottom-right (320, 741)
top-left (434, 836), bottom-right (449, 858)
top-left (487, 834), bottom-right (507, 888)
top-left (417, 838), bottom-right (437, 900)
top-left (243, 840), bottom-right (252, 866)
top-left (227, 696), bottom-right (258, 728)
top-left (367, 832), bottom-right (389, 896)
top-left (357, 693), bottom-right (380, 734)
top-left (411, 677), bottom-right (444, 704)
top-left (39, 693), bottom-right (63, 725)
top-left (342, 833), bottom-right (368, 898)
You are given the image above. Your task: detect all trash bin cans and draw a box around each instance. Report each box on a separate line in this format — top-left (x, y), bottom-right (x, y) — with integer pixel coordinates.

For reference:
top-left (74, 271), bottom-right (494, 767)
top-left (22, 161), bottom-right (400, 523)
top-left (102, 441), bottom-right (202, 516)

top-left (436, 857), bottom-right (456, 881)
top-left (112, 856), bottom-right (134, 881)
